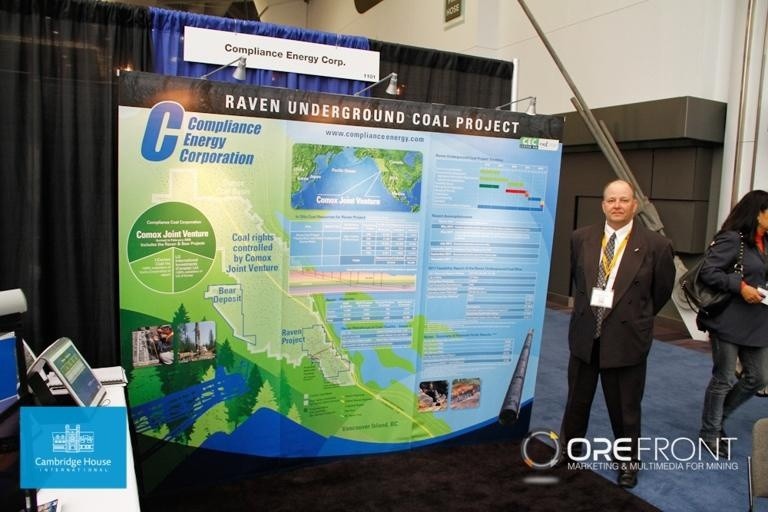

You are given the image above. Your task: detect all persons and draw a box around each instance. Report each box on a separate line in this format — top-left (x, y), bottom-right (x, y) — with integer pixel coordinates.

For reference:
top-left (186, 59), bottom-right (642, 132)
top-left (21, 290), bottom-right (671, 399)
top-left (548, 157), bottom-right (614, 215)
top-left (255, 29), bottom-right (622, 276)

top-left (694, 189), bottom-right (768, 460)
top-left (145, 326), bottom-right (174, 364)
top-left (556, 179), bottom-right (677, 489)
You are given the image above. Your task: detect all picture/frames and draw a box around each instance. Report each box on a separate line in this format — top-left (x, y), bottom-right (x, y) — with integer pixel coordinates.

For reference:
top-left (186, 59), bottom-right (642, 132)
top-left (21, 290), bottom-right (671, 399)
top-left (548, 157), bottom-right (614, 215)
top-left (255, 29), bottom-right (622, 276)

top-left (443, 0), bottom-right (465, 28)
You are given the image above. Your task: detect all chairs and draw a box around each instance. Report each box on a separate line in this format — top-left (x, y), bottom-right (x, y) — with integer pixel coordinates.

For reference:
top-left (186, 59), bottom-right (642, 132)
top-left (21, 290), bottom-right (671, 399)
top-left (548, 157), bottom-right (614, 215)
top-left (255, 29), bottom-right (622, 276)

top-left (747, 419), bottom-right (768, 512)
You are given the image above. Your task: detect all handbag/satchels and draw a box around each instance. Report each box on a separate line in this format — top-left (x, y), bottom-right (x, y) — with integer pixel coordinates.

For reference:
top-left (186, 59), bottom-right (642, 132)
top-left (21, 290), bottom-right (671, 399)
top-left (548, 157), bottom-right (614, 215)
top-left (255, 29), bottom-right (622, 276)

top-left (677, 226), bottom-right (748, 319)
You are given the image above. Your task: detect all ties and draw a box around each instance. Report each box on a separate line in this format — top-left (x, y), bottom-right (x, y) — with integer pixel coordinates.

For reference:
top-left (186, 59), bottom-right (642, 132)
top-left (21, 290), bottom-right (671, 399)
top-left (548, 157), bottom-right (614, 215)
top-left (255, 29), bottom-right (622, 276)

top-left (590, 232), bottom-right (618, 342)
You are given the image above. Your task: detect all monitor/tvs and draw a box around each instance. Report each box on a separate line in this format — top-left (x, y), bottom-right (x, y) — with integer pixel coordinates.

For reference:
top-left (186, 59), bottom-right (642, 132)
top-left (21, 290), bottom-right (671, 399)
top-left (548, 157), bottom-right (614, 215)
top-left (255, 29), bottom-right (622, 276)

top-left (27, 338), bottom-right (106, 407)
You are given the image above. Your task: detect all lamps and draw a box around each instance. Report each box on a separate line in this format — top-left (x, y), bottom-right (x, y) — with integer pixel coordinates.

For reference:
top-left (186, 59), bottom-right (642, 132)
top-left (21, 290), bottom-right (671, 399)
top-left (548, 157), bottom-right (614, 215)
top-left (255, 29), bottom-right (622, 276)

top-left (354, 72), bottom-right (397, 97)
top-left (497, 97), bottom-right (536, 115)
top-left (201, 56), bottom-right (245, 81)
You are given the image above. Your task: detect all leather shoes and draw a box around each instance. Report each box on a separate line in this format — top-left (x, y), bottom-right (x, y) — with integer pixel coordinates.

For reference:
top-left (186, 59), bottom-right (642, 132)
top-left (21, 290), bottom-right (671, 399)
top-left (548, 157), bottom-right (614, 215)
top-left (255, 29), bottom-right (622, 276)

top-left (616, 460), bottom-right (640, 488)
top-left (719, 426), bottom-right (733, 448)
top-left (545, 450), bottom-right (583, 471)
top-left (699, 430), bottom-right (728, 459)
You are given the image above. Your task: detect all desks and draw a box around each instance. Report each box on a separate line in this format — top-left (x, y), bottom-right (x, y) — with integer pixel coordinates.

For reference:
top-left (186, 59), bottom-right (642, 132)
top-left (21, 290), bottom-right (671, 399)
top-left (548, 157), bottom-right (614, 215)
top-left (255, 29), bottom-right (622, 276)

top-left (25, 363), bottom-right (142, 512)
